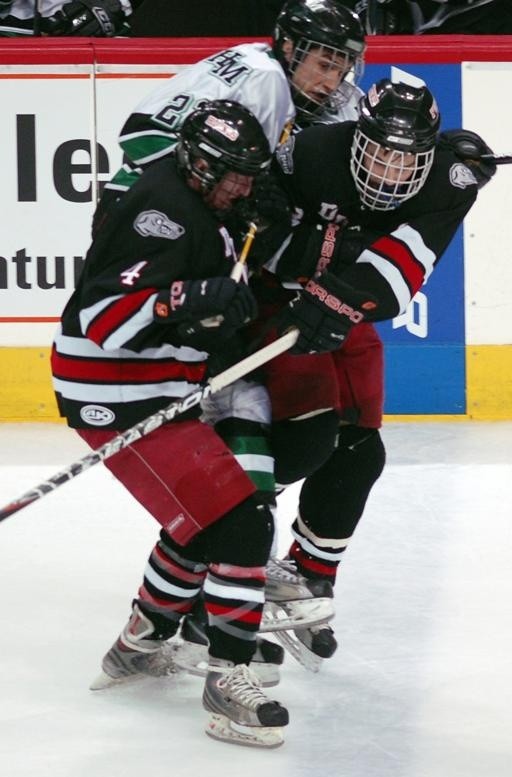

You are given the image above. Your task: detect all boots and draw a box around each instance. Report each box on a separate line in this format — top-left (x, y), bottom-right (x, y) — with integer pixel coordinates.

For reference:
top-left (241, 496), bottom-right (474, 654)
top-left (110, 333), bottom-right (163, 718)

top-left (203, 663), bottom-right (288, 727)
top-left (102, 604), bottom-right (176, 679)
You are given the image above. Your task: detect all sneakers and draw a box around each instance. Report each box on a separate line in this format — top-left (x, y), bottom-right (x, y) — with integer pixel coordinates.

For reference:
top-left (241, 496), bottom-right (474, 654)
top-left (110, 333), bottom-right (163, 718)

top-left (181, 556), bottom-right (336, 664)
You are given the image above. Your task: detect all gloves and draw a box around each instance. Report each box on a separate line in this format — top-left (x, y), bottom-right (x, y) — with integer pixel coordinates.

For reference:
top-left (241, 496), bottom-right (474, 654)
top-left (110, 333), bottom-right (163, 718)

top-left (153, 277), bottom-right (259, 325)
top-left (277, 269), bottom-right (380, 355)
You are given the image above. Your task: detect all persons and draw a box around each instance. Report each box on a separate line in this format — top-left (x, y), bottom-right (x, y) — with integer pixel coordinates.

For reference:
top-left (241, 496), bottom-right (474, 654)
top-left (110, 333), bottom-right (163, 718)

top-left (1, 1), bottom-right (511, 35)
top-left (80, 0), bottom-right (496, 510)
top-left (47, 95), bottom-right (296, 729)
top-left (178, 72), bottom-right (497, 668)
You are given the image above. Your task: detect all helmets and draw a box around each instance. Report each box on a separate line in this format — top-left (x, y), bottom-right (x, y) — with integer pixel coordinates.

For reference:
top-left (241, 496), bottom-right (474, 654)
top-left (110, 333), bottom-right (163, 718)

top-left (350, 79), bottom-right (440, 212)
top-left (273, 0), bottom-right (365, 121)
top-left (176, 100), bottom-right (273, 221)
